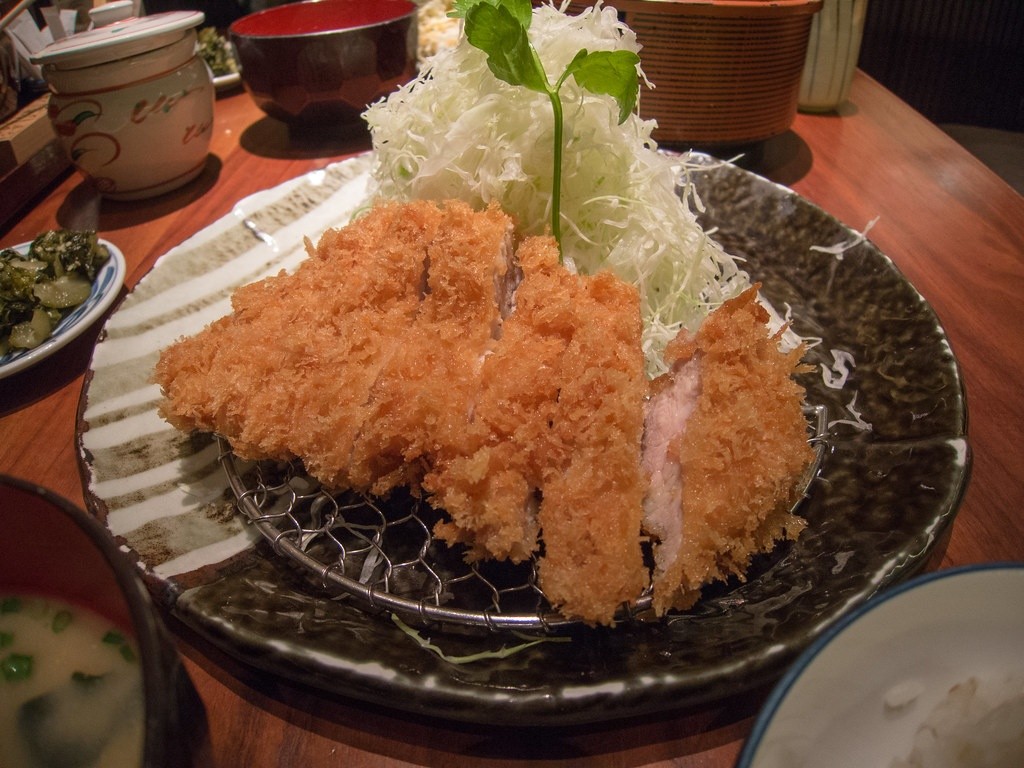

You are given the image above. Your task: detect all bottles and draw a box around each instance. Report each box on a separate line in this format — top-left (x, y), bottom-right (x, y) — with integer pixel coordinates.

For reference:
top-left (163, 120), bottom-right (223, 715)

top-left (26, 0), bottom-right (217, 202)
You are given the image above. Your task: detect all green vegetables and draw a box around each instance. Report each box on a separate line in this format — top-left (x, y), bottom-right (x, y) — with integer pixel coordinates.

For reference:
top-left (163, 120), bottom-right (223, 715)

top-left (445, 1), bottom-right (638, 270)
top-left (0, 226), bottom-right (112, 355)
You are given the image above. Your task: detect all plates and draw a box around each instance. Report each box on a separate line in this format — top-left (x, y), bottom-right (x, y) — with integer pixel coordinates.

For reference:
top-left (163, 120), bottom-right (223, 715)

top-left (1, 239), bottom-right (123, 374)
top-left (734, 562), bottom-right (1024, 768)
top-left (77, 147), bottom-right (970, 731)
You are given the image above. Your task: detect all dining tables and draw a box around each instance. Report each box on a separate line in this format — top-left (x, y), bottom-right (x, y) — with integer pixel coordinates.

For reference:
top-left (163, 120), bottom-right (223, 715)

top-left (0, 66), bottom-right (1024, 768)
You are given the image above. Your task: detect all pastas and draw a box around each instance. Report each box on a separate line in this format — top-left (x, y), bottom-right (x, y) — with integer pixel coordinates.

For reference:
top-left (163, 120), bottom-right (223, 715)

top-left (414, 1), bottom-right (463, 61)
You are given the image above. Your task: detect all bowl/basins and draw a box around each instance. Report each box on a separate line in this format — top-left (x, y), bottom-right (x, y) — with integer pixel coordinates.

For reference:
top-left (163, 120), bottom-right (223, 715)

top-left (227, 0), bottom-right (421, 139)
top-left (1, 468), bottom-right (195, 768)
